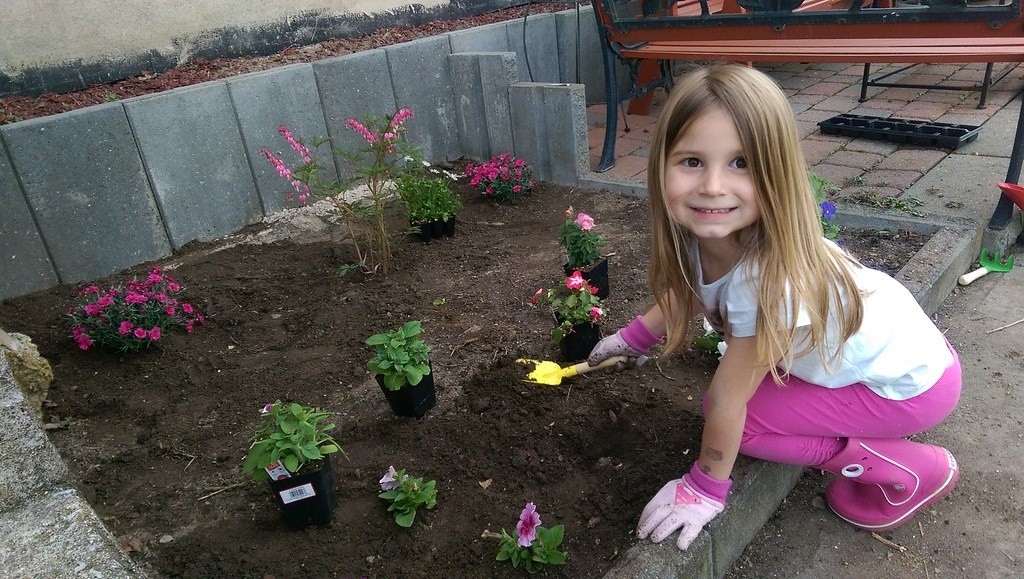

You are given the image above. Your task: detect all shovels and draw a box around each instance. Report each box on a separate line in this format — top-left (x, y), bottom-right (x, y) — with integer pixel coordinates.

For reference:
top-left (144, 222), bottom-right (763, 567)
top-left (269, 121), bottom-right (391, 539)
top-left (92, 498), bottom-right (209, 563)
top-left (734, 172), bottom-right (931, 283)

top-left (516, 352), bottom-right (629, 386)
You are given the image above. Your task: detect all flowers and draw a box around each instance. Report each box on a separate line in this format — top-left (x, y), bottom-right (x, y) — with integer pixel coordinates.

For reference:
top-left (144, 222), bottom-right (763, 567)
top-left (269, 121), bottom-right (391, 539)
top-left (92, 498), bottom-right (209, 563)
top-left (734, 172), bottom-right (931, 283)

top-left (558, 205), bottom-right (606, 268)
top-left (242, 398), bottom-right (350, 484)
top-left (532, 270), bottom-right (607, 341)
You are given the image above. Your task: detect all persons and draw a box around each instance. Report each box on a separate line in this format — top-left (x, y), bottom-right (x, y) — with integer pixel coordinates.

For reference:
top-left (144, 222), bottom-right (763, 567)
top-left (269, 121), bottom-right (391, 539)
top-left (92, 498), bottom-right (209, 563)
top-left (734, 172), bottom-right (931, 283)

top-left (588, 64), bottom-right (962, 551)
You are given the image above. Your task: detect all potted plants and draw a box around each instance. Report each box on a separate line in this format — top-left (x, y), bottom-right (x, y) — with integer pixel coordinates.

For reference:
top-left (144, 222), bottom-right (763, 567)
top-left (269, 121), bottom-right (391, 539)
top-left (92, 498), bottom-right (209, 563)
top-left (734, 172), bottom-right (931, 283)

top-left (365, 321), bottom-right (436, 419)
top-left (396, 173), bottom-right (461, 239)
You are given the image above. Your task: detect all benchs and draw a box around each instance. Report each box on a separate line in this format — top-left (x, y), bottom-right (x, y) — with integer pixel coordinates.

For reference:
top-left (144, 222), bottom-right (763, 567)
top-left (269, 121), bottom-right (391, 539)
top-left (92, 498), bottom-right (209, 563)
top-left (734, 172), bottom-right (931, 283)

top-left (588, 1), bottom-right (1024, 230)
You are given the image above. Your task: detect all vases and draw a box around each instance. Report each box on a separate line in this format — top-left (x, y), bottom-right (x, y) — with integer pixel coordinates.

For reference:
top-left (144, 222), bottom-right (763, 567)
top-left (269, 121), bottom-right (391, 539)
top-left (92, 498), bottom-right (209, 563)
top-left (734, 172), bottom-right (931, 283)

top-left (269, 451), bottom-right (338, 529)
top-left (563, 256), bottom-right (608, 301)
top-left (555, 320), bottom-right (602, 361)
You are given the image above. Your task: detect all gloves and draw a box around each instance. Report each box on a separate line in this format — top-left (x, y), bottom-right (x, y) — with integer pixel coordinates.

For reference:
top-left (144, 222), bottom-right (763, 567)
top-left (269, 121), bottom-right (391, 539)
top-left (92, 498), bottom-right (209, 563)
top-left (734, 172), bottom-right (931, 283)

top-left (589, 315), bottom-right (663, 374)
top-left (635, 461), bottom-right (731, 550)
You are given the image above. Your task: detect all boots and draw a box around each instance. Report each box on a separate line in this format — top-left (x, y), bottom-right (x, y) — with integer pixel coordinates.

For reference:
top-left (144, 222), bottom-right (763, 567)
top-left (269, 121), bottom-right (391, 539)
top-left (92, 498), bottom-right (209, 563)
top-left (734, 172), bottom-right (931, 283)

top-left (813, 438), bottom-right (959, 531)
